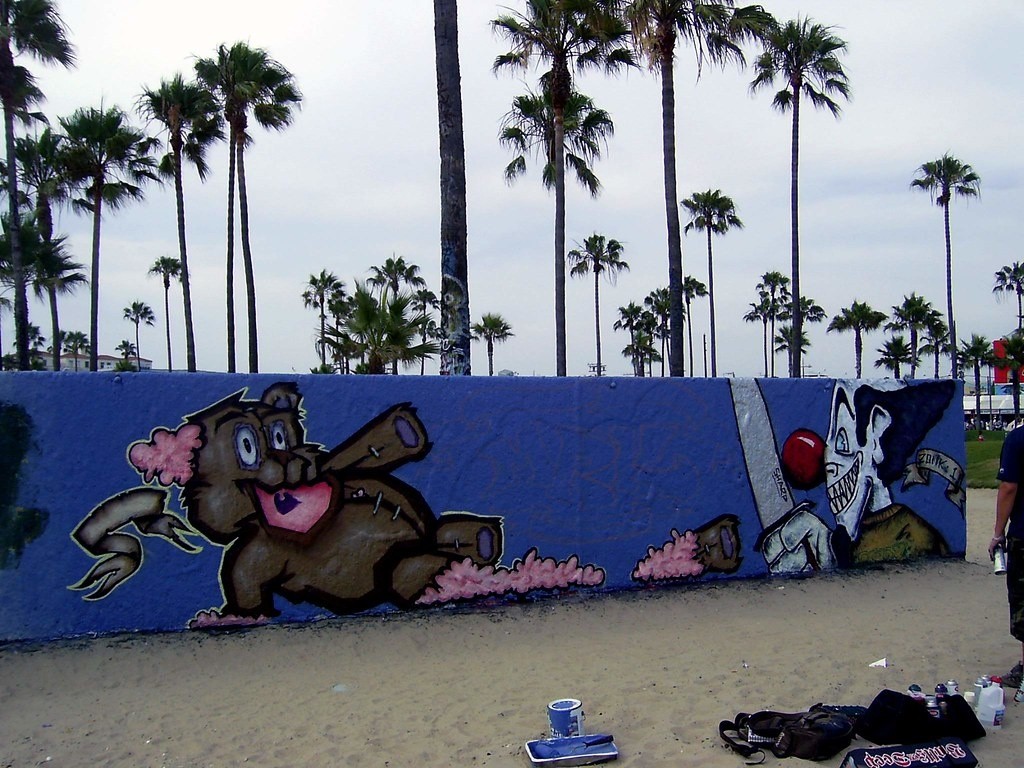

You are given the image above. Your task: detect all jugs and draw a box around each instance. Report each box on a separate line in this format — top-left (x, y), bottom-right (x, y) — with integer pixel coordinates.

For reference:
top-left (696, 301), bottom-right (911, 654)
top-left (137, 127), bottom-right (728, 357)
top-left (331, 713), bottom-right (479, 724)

top-left (975, 676), bottom-right (1004, 730)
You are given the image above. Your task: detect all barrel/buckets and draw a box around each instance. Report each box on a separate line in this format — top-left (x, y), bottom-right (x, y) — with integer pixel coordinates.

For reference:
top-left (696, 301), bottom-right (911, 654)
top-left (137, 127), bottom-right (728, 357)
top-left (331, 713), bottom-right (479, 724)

top-left (547, 699), bottom-right (586, 738)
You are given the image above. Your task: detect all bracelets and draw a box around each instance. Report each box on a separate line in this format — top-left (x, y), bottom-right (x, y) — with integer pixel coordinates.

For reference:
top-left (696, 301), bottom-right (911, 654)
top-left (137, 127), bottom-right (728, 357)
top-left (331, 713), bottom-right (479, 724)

top-left (993, 536), bottom-right (1004, 540)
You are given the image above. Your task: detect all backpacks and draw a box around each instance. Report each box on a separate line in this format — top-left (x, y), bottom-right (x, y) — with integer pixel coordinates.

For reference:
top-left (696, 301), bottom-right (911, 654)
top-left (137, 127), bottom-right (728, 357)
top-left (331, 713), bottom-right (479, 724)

top-left (719, 701), bottom-right (853, 765)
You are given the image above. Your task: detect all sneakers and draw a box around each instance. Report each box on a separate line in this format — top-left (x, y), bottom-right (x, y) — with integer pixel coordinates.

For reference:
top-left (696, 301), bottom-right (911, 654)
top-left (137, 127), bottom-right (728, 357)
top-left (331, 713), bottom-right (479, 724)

top-left (1001, 661), bottom-right (1024, 688)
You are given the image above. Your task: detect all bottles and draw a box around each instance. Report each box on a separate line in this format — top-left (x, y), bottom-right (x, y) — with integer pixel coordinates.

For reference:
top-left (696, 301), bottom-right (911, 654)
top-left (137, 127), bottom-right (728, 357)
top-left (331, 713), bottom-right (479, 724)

top-left (992, 545), bottom-right (1007, 575)
top-left (909, 675), bottom-right (992, 717)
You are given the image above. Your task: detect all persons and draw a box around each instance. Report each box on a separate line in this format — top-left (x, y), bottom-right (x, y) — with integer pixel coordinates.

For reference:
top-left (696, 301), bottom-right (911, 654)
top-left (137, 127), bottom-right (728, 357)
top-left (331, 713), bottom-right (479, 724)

top-left (988, 424), bottom-right (1024, 688)
top-left (964, 415), bottom-right (1024, 441)
